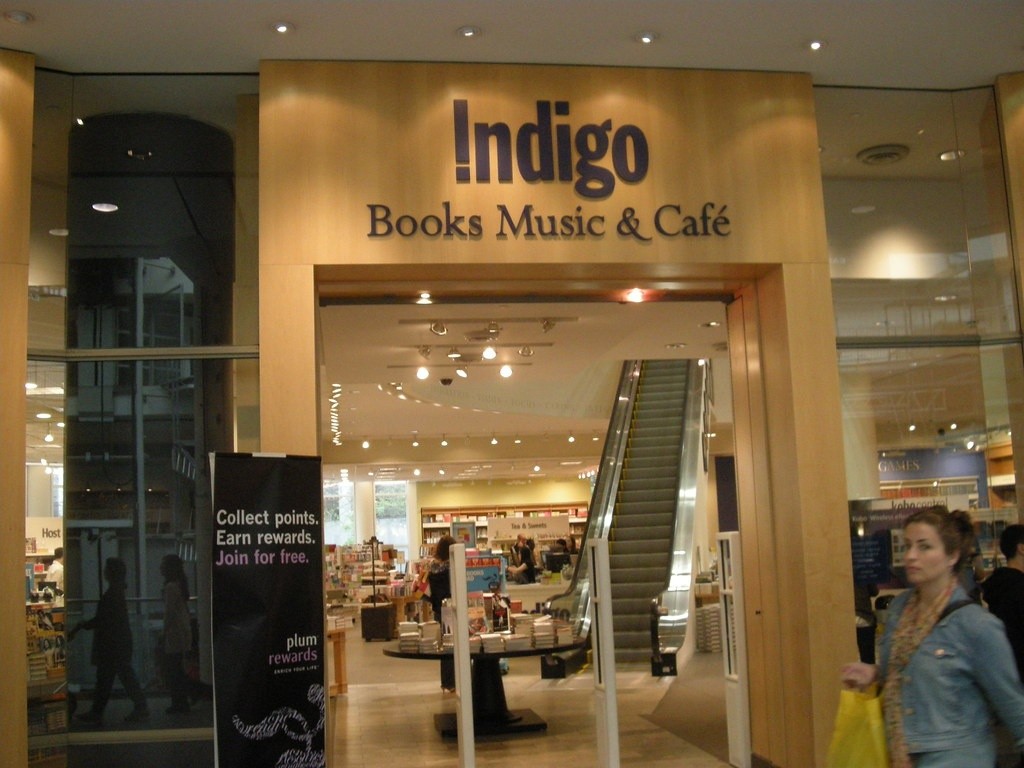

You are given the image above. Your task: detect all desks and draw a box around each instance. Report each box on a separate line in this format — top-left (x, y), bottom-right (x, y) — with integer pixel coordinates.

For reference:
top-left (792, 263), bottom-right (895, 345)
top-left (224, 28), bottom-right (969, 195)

top-left (327, 627), bottom-right (355, 696)
top-left (382, 634), bottom-right (587, 737)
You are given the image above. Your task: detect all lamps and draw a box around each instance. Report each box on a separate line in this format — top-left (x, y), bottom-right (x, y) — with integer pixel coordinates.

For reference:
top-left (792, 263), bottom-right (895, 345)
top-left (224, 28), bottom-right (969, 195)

top-left (518, 345), bottom-right (534, 356)
top-left (447, 347), bottom-right (461, 358)
top-left (539, 321), bottom-right (554, 333)
top-left (418, 346), bottom-right (431, 359)
top-left (430, 322), bottom-right (447, 335)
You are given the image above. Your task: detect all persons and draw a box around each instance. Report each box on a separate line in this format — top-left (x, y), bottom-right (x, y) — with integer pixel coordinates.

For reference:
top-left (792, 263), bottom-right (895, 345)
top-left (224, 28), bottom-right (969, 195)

top-left (428, 534), bottom-right (457, 693)
top-left (45, 547), bottom-right (63, 593)
top-left (74, 557), bottom-right (149, 726)
top-left (841, 508), bottom-right (1024, 767)
top-left (153, 555), bottom-right (193, 716)
top-left (949, 511), bottom-right (984, 604)
top-left (505, 536), bottom-right (575, 584)
top-left (983, 524), bottom-right (1024, 683)
top-left (866, 530), bottom-right (908, 609)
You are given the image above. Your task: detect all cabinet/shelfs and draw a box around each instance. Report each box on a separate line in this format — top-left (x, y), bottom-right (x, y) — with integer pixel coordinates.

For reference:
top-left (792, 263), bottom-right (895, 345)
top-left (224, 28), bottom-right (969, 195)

top-left (421, 501), bottom-right (588, 557)
top-left (323, 544), bottom-right (393, 616)
